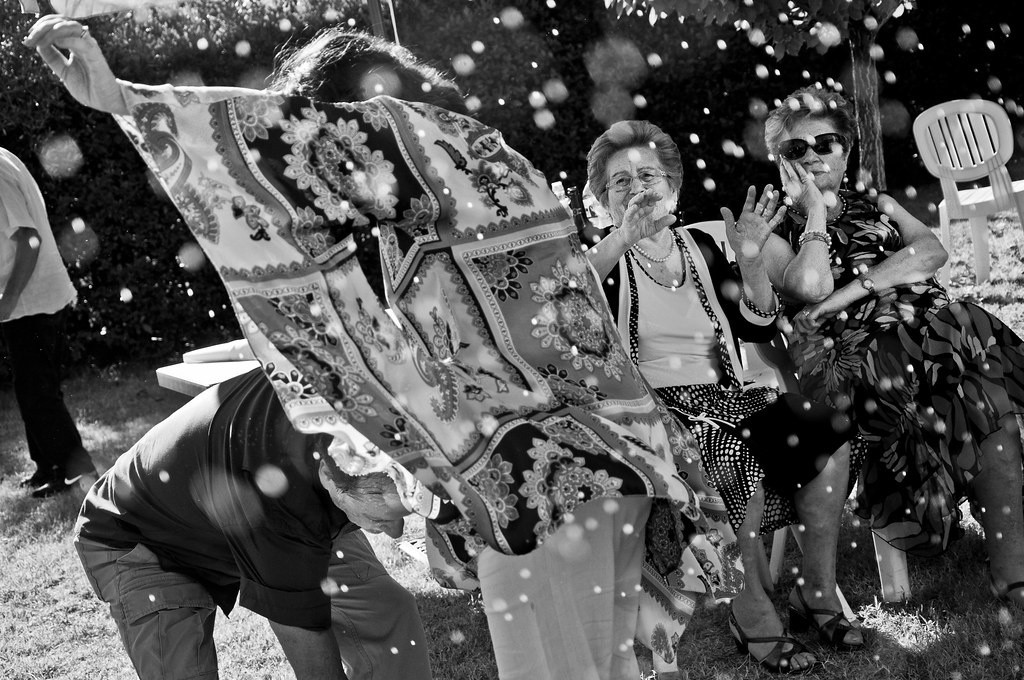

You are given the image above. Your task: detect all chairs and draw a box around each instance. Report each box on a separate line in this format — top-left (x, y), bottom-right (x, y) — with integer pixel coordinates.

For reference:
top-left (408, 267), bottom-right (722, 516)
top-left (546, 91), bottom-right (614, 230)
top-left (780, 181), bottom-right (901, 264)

top-left (645, 219), bottom-right (911, 677)
top-left (912, 96), bottom-right (1024, 294)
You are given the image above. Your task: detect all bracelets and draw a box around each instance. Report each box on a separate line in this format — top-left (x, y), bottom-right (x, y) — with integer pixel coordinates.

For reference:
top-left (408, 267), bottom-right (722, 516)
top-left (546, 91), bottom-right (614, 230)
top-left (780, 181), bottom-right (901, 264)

top-left (799, 230), bottom-right (832, 249)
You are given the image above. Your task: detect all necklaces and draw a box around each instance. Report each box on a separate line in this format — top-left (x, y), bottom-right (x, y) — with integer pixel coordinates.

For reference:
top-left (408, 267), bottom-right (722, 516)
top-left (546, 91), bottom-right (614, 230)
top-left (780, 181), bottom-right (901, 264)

top-left (634, 230), bottom-right (676, 263)
top-left (786, 194), bottom-right (847, 223)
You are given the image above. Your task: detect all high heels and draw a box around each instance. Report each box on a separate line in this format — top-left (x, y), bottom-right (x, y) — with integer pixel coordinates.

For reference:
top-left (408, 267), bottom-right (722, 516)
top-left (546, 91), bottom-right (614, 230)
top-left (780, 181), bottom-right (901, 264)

top-left (729, 612), bottom-right (820, 676)
top-left (787, 583), bottom-right (864, 650)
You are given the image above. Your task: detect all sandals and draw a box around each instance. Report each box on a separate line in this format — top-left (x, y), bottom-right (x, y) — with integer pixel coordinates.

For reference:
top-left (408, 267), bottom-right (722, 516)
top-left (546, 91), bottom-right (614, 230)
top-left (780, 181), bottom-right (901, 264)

top-left (980, 562), bottom-right (1024, 613)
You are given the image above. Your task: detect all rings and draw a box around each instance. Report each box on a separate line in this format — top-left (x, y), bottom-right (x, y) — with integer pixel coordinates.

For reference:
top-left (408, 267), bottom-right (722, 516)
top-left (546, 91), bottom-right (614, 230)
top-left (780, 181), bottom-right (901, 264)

top-left (80, 26), bottom-right (89, 38)
top-left (803, 310), bottom-right (809, 316)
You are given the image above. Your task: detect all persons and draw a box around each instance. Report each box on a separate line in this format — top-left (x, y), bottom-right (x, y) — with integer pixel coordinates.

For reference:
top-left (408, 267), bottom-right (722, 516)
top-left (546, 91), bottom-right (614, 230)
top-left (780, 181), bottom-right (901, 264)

top-left (0, 147), bottom-right (100, 499)
top-left (583, 120), bottom-right (868, 677)
top-left (73, 365), bottom-right (434, 680)
top-left (22, 12), bottom-right (745, 680)
top-left (757, 84), bottom-right (1024, 605)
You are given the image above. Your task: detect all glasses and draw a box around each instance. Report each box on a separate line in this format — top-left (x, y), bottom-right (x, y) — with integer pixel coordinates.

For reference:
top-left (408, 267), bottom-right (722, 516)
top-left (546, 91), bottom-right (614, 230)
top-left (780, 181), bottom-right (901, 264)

top-left (606, 168), bottom-right (666, 191)
top-left (777, 132), bottom-right (849, 161)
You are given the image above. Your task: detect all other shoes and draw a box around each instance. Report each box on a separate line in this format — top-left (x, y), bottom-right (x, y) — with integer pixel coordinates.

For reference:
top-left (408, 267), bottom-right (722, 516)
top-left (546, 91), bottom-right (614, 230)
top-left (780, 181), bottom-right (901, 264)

top-left (20, 462), bottom-right (99, 498)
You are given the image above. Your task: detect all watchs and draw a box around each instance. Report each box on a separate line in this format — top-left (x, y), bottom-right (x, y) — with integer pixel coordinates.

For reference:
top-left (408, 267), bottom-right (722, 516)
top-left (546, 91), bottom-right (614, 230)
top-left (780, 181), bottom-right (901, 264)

top-left (858, 274), bottom-right (876, 293)
